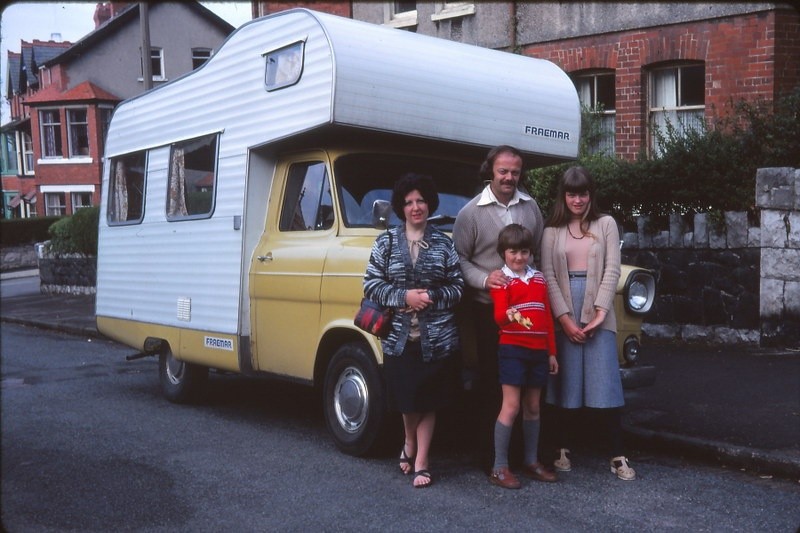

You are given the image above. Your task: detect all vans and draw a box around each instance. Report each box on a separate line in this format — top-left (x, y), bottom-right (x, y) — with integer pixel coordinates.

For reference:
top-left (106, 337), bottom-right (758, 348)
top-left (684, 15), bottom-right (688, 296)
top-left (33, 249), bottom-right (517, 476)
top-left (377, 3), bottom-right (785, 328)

top-left (96, 6), bottom-right (661, 456)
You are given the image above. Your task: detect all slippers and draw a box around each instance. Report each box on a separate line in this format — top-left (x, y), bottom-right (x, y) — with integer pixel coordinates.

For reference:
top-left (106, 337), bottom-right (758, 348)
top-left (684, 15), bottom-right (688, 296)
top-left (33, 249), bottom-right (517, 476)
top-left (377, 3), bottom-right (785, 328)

top-left (413, 469), bottom-right (433, 488)
top-left (398, 445), bottom-right (416, 474)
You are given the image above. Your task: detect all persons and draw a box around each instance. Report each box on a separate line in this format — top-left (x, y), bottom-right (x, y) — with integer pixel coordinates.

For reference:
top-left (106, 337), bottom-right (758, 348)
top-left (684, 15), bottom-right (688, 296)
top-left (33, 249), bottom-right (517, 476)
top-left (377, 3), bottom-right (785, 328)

top-left (363, 174), bottom-right (464, 487)
top-left (541, 166), bottom-right (636, 481)
top-left (489, 223), bottom-right (559, 488)
top-left (452, 146), bottom-right (544, 475)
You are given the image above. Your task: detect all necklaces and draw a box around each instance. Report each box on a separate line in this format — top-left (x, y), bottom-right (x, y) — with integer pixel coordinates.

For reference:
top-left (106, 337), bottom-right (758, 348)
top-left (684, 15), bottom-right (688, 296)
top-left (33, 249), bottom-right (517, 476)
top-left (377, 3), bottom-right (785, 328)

top-left (567, 220), bottom-right (590, 239)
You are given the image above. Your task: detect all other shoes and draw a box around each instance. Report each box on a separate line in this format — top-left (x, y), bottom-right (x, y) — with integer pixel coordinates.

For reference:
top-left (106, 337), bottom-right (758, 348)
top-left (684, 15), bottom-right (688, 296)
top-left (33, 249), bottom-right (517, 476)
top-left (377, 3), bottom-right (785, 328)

top-left (491, 467), bottom-right (521, 488)
top-left (553, 447), bottom-right (573, 472)
top-left (610, 454), bottom-right (636, 482)
top-left (520, 462), bottom-right (560, 483)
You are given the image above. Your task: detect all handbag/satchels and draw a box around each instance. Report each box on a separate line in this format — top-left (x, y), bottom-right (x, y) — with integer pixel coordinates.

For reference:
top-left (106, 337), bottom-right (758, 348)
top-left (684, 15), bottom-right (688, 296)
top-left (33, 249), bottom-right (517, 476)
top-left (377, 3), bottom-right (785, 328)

top-left (354, 232), bottom-right (394, 340)
